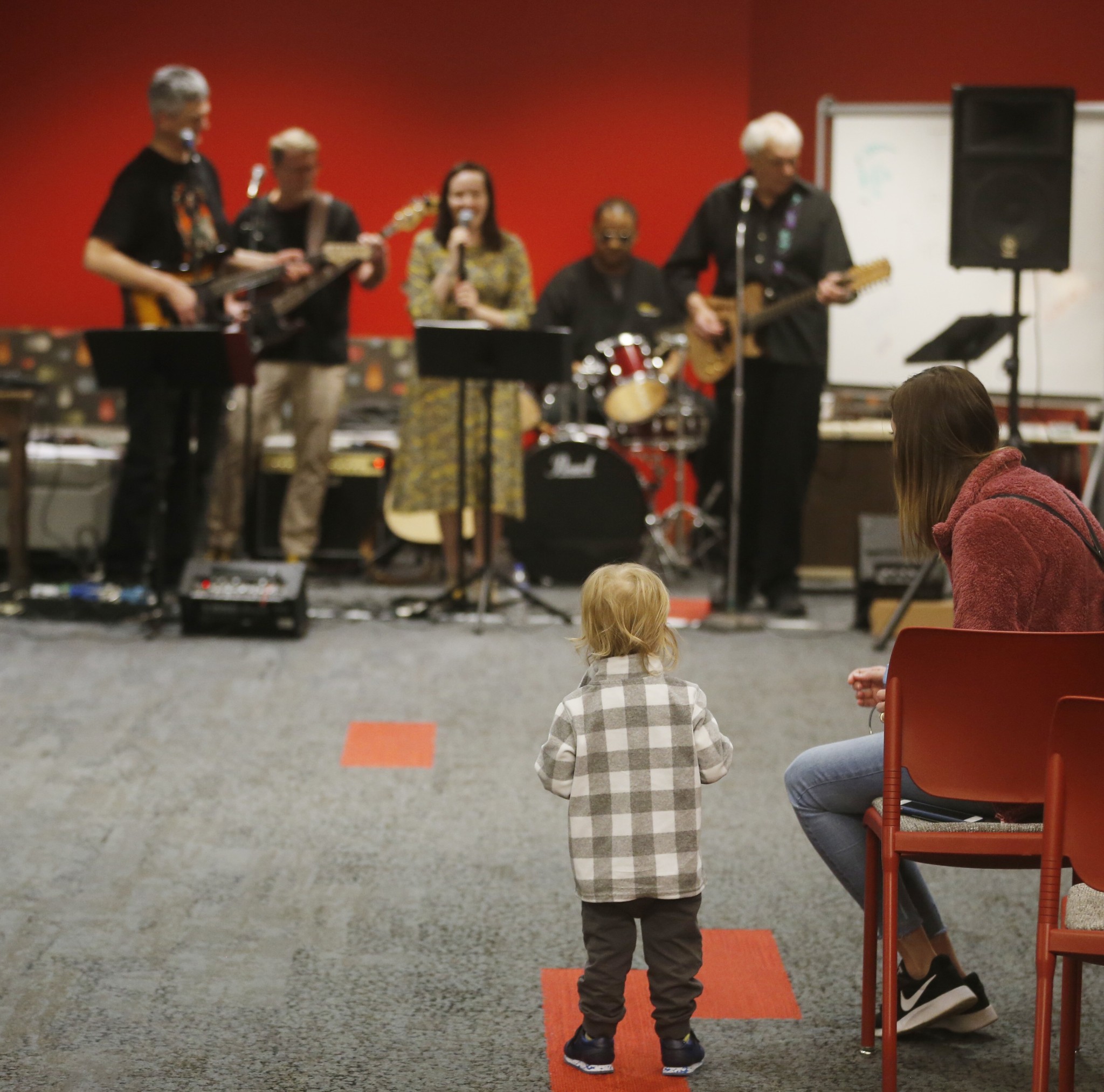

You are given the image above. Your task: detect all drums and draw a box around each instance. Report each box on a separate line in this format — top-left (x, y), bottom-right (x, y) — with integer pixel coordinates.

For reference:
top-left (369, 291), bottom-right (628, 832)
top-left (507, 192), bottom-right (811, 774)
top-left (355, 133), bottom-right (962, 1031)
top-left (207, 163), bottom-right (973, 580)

top-left (611, 404), bottom-right (715, 453)
top-left (586, 334), bottom-right (671, 423)
top-left (536, 383), bottom-right (615, 439)
top-left (496, 434), bottom-right (699, 596)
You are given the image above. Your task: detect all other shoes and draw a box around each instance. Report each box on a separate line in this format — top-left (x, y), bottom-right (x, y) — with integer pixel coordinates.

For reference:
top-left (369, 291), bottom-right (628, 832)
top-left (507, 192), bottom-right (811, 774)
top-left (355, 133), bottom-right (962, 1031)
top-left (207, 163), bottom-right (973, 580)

top-left (763, 586), bottom-right (805, 616)
top-left (711, 581), bottom-right (752, 611)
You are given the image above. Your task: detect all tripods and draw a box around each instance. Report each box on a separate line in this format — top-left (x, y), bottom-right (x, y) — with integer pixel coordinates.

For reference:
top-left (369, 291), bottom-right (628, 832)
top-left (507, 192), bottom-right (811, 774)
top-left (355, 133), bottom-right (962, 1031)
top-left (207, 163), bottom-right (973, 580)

top-left (871, 270), bottom-right (1043, 651)
top-left (414, 324), bottom-right (578, 632)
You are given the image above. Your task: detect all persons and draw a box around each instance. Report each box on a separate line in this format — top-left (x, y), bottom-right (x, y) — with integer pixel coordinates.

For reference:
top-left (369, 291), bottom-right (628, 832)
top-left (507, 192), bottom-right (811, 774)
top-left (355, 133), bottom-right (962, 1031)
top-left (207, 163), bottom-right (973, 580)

top-left (523, 196), bottom-right (688, 426)
top-left (662, 111), bottom-right (857, 630)
top-left (784, 364), bottom-right (1104, 1034)
top-left (207, 129), bottom-right (387, 563)
top-left (392, 160), bottom-right (535, 623)
top-left (536, 563), bottom-right (734, 1075)
top-left (83, 64), bottom-right (314, 624)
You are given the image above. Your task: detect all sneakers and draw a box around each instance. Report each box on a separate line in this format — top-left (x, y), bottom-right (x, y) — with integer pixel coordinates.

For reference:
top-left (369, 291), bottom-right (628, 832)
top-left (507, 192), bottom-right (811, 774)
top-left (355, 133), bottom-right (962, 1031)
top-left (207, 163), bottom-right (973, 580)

top-left (874, 953), bottom-right (977, 1036)
top-left (661, 1029), bottom-right (705, 1077)
top-left (930, 971), bottom-right (997, 1034)
top-left (564, 1023), bottom-right (616, 1074)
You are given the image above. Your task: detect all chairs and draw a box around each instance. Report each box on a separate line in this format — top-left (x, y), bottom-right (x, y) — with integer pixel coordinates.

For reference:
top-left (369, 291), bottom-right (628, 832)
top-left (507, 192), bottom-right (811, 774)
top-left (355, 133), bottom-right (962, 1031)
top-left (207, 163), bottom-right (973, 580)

top-left (862, 626), bottom-right (1104, 1092)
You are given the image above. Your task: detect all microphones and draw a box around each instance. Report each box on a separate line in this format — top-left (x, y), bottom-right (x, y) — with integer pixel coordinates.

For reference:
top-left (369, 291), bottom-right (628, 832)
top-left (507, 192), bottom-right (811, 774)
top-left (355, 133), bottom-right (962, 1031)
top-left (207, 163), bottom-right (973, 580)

top-left (738, 175), bottom-right (758, 213)
top-left (179, 127), bottom-right (197, 157)
top-left (458, 205), bottom-right (474, 231)
top-left (246, 163), bottom-right (265, 200)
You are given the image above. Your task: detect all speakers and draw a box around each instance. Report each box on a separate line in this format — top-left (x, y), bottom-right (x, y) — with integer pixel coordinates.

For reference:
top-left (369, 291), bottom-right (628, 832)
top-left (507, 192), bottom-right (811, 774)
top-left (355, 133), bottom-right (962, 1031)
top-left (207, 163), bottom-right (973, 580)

top-left (950, 84), bottom-right (1072, 276)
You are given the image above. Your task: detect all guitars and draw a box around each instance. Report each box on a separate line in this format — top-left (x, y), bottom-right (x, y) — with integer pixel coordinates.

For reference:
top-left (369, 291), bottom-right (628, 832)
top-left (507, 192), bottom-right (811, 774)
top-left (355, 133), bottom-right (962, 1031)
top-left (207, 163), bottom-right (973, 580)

top-left (129, 237), bottom-right (373, 332)
top-left (679, 259), bottom-right (892, 384)
top-left (236, 190), bottom-right (445, 341)
